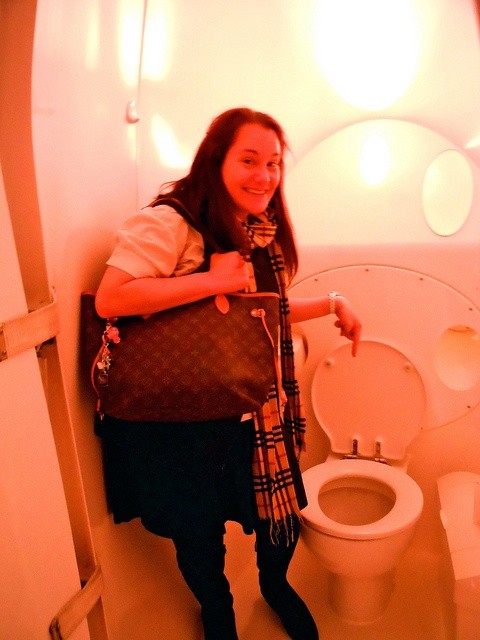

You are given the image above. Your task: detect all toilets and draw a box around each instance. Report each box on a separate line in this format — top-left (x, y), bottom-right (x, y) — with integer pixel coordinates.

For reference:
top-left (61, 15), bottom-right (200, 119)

top-left (300, 340), bottom-right (428, 623)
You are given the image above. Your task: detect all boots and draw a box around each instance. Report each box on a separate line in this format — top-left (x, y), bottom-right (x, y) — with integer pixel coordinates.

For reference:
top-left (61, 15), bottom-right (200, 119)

top-left (175, 545), bottom-right (239, 640)
top-left (257, 542), bottom-right (320, 640)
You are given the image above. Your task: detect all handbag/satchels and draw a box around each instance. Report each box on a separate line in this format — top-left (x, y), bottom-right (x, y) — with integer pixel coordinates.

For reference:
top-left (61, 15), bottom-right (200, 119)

top-left (77, 199), bottom-right (281, 422)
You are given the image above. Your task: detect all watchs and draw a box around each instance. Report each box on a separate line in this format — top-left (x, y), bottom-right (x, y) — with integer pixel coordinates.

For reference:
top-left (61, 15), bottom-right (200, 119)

top-left (328, 291), bottom-right (340, 316)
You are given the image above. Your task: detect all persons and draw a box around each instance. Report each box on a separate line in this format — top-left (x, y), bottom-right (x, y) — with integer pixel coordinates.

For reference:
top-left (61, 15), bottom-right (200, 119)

top-left (94, 107), bottom-right (361, 639)
